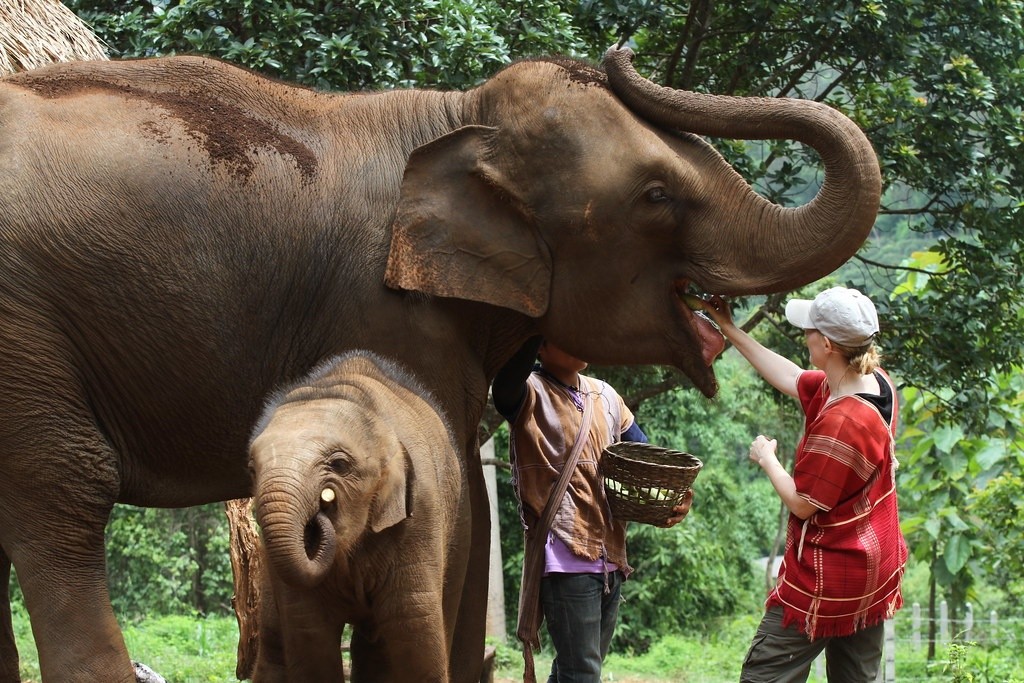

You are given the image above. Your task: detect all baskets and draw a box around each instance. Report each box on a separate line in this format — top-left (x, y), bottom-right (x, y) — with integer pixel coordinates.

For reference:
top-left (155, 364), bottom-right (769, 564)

top-left (603, 442), bottom-right (703, 526)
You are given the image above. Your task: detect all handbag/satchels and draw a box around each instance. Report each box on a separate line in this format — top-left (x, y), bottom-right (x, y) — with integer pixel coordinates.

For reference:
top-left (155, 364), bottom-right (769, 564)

top-left (517, 529), bottom-right (546, 652)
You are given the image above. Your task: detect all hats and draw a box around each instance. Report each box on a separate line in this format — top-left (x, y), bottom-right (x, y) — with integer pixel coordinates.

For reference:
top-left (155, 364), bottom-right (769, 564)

top-left (785, 286), bottom-right (879, 347)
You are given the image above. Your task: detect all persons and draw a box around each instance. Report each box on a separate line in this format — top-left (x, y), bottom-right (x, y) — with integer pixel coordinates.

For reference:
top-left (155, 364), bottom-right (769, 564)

top-left (491, 334), bottom-right (694, 683)
top-left (697, 287), bottom-right (909, 683)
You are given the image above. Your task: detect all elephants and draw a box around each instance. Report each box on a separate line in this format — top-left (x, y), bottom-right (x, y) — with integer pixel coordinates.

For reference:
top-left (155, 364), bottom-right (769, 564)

top-left (0, 44), bottom-right (882, 683)
